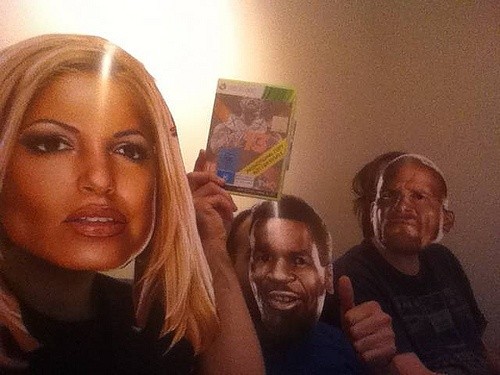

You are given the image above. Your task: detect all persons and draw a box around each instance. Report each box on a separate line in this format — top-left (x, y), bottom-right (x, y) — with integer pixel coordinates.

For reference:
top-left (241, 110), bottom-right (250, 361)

top-left (0, 33), bottom-right (218, 375)
top-left (187, 149), bottom-right (493, 375)
